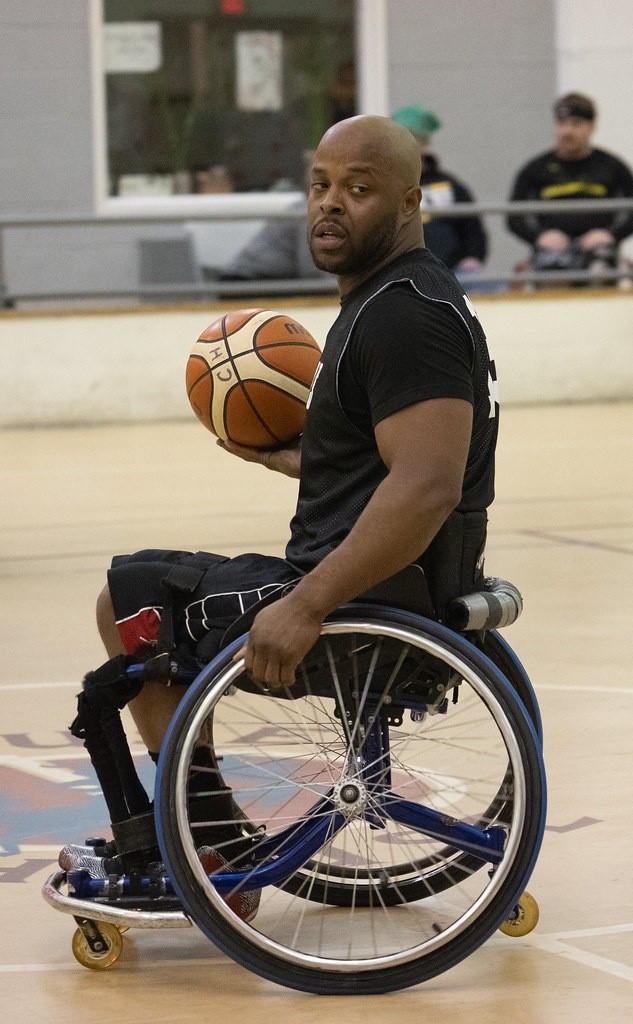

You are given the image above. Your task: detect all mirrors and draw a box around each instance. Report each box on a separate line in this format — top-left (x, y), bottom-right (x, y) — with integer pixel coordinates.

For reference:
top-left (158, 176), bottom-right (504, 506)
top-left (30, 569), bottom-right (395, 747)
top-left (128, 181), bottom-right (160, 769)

top-left (89, 1), bottom-right (392, 219)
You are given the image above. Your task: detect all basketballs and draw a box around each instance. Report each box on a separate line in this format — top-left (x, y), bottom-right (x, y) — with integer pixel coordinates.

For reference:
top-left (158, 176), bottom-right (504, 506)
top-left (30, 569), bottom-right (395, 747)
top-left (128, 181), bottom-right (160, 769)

top-left (183, 307), bottom-right (324, 450)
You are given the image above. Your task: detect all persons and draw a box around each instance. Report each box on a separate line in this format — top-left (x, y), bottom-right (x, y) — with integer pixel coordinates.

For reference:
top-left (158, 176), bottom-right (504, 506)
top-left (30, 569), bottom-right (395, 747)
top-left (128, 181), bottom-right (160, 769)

top-left (387, 105), bottom-right (490, 268)
top-left (53, 111), bottom-right (501, 924)
top-left (506, 93), bottom-right (633, 290)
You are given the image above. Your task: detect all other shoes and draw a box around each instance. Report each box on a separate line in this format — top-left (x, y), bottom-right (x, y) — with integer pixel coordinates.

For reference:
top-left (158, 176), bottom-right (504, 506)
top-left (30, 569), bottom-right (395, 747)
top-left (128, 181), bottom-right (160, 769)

top-left (69, 824), bottom-right (265, 924)
top-left (57, 841), bottom-right (116, 872)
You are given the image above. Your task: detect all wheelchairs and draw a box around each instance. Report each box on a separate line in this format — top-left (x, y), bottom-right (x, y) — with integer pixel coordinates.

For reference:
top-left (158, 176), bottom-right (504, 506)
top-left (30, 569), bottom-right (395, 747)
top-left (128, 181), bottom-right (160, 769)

top-left (39, 511), bottom-right (550, 997)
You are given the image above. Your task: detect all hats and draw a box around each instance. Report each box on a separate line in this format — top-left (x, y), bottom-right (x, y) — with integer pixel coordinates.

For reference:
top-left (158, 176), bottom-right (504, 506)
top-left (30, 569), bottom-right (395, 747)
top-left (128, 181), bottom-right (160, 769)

top-left (391, 103), bottom-right (440, 141)
